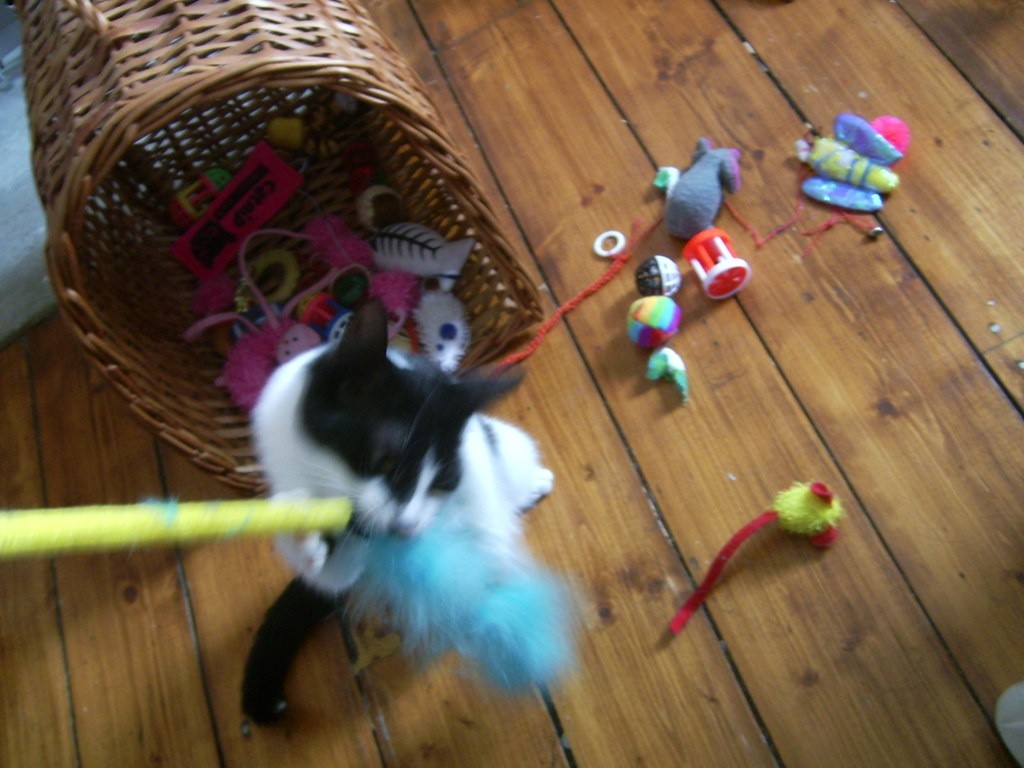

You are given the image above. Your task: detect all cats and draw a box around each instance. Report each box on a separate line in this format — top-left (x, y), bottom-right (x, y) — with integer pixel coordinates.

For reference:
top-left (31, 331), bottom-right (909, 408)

top-left (240, 292), bottom-right (560, 728)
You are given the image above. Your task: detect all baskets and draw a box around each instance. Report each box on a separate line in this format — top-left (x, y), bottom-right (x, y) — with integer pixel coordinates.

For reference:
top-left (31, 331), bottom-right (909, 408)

top-left (16, 0), bottom-right (544, 492)
